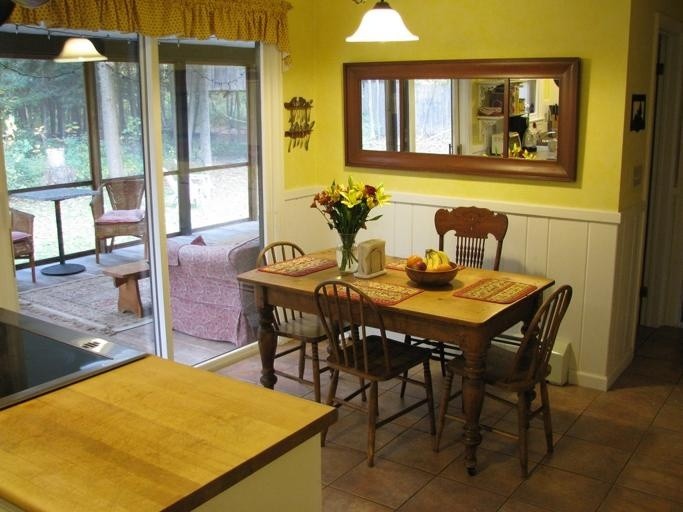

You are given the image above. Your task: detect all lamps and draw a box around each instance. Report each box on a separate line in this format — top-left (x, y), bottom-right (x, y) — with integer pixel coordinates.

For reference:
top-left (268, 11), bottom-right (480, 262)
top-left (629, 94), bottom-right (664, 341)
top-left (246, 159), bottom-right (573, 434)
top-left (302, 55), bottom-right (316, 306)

top-left (345, 0), bottom-right (420, 43)
top-left (53, 35), bottom-right (109, 64)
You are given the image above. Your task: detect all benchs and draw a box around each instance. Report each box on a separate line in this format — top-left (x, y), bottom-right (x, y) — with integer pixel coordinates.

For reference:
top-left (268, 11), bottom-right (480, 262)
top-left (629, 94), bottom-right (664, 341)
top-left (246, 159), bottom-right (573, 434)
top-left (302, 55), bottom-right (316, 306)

top-left (105, 261), bottom-right (150, 318)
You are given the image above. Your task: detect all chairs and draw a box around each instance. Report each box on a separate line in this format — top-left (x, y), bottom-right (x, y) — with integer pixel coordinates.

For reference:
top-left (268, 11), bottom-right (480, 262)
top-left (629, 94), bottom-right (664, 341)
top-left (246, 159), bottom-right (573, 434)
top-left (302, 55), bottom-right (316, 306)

top-left (166, 232), bottom-right (258, 345)
top-left (89, 179), bottom-right (149, 264)
top-left (9, 206), bottom-right (38, 283)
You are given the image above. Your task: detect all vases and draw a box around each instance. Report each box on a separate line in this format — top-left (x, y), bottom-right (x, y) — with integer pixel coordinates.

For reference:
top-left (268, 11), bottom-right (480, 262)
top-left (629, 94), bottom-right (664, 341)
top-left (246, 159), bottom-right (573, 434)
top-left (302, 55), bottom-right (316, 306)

top-left (336, 231), bottom-right (361, 272)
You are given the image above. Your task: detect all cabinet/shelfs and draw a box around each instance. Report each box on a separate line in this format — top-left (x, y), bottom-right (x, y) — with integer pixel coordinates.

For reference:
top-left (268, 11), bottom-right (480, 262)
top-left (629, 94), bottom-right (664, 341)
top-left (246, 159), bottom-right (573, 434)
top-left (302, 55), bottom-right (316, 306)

top-left (476, 77), bottom-right (528, 119)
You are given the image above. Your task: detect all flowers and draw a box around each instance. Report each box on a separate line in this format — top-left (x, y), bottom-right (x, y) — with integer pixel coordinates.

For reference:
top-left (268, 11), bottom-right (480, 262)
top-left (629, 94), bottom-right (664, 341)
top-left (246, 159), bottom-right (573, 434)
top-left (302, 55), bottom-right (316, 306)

top-left (311, 178), bottom-right (390, 270)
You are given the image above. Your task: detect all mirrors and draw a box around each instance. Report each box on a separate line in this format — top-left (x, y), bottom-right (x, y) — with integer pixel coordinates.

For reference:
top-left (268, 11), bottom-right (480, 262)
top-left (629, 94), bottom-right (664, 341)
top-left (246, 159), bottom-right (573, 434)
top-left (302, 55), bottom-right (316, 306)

top-left (340, 56), bottom-right (582, 184)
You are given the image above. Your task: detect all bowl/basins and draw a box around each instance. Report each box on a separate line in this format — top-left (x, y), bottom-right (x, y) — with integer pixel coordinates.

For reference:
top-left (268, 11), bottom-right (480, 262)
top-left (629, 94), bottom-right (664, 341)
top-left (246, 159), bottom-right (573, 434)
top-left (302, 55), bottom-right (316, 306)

top-left (404, 262), bottom-right (458, 285)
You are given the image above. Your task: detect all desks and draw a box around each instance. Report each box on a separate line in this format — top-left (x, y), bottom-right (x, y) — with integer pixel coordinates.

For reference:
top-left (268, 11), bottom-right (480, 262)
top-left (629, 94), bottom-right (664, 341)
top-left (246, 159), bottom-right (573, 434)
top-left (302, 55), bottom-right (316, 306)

top-left (13, 188), bottom-right (101, 275)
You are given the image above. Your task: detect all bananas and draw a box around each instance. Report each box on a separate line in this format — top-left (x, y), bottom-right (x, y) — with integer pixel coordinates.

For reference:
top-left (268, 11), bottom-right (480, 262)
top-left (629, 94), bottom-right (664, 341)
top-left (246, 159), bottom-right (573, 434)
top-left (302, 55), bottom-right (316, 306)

top-left (422, 249), bottom-right (450, 272)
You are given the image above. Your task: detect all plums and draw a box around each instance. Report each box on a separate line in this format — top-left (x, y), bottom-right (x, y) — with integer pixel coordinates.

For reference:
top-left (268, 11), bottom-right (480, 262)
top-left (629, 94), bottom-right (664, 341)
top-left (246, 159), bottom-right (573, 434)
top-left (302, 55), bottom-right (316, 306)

top-left (412, 261), bottom-right (426, 270)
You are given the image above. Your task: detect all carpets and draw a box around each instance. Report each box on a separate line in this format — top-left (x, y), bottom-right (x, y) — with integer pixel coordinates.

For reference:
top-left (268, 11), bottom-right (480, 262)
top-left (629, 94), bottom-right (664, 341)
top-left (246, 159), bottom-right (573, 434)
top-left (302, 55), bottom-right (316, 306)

top-left (16, 263), bottom-right (151, 339)
